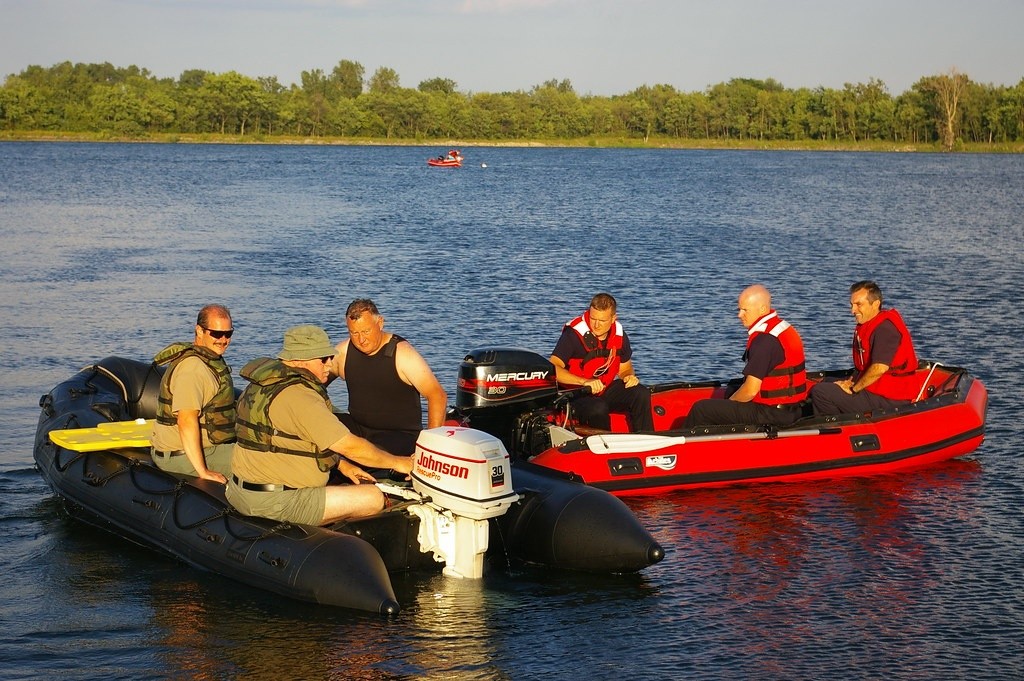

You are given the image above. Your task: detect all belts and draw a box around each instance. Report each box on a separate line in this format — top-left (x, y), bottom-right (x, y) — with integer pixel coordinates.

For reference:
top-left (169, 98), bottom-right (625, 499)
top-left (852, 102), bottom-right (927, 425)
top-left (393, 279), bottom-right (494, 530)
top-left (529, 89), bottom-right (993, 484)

top-left (773, 403), bottom-right (800, 409)
top-left (232, 474), bottom-right (301, 492)
top-left (151, 446), bottom-right (186, 458)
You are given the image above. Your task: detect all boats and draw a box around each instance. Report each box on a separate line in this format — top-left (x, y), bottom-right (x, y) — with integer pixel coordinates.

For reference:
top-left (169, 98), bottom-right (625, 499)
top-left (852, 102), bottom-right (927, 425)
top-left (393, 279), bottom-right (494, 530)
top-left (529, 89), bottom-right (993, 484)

top-left (32, 354), bottom-right (667, 628)
top-left (454, 343), bottom-right (987, 501)
top-left (427, 153), bottom-right (466, 168)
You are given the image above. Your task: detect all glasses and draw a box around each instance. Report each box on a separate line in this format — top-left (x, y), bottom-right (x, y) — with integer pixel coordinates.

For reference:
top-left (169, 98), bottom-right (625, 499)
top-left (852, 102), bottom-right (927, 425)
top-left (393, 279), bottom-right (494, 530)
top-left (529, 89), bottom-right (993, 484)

top-left (197, 323), bottom-right (235, 339)
top-left (315, 355), bottom-right (334, 363)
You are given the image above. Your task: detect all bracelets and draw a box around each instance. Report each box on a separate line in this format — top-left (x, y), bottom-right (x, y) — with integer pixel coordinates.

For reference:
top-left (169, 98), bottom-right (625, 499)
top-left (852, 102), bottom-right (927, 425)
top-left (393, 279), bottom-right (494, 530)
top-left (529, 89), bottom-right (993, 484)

top-left (850, 385), bottom-right (856, 394)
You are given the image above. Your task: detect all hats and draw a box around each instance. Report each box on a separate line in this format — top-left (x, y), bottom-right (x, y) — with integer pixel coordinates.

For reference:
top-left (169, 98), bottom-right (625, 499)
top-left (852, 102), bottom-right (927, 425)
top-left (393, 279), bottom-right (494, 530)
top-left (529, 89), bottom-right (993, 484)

top-left (275, 324), bottom-right (340, 361)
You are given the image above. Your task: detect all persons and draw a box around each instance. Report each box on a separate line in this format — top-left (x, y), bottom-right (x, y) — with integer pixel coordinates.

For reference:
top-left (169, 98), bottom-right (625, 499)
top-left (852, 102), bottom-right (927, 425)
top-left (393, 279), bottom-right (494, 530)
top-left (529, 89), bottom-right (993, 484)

top-left (446, 149), bottom-right (463, 162)
top-left (681, 285), bottom-right (806, 430)
top-left (811, 281), bottom-right (918, 418)
top-left (150, 305), bottom-right (238, 484)
top-left (224, 325), bottom-right (413, 527)
top-left (322, 298), bottom-right (447, 487)
top-left (549, 293), bottom-right (654, 433)
